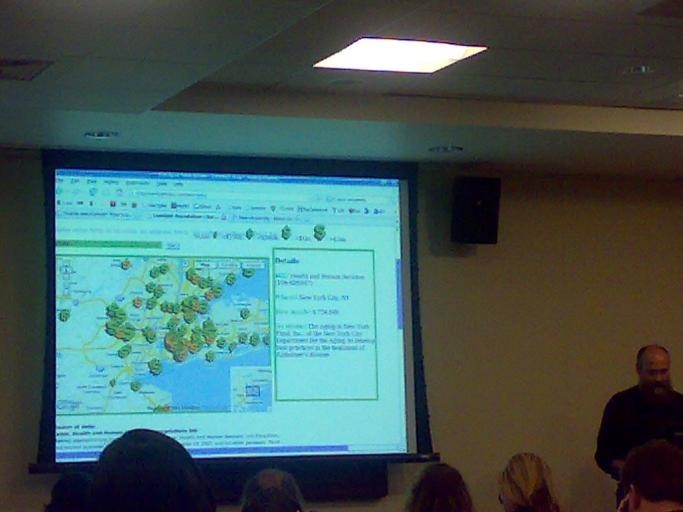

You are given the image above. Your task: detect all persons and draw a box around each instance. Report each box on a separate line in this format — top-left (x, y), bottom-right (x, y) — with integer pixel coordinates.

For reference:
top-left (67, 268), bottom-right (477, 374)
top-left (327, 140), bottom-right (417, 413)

top-left (593, 345), bottom-right (682, 511)
top-left (42, 475), bottom-right (90, 511)
top-left (239, 469), bottom-right (306, 511)
top-left (497, 452), bottom-right (559, 511)
top-left (404, 463), bottom-right (472, 512)
top-left (86, 429), bottom-right (217, 511)
top-left (616, 439), bottom-right (682, 510)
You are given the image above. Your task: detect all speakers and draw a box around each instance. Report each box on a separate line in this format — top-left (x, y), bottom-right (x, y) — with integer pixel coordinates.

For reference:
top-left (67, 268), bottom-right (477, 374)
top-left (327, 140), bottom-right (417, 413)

top-left (450, 176), bottom-right (503, 245)
